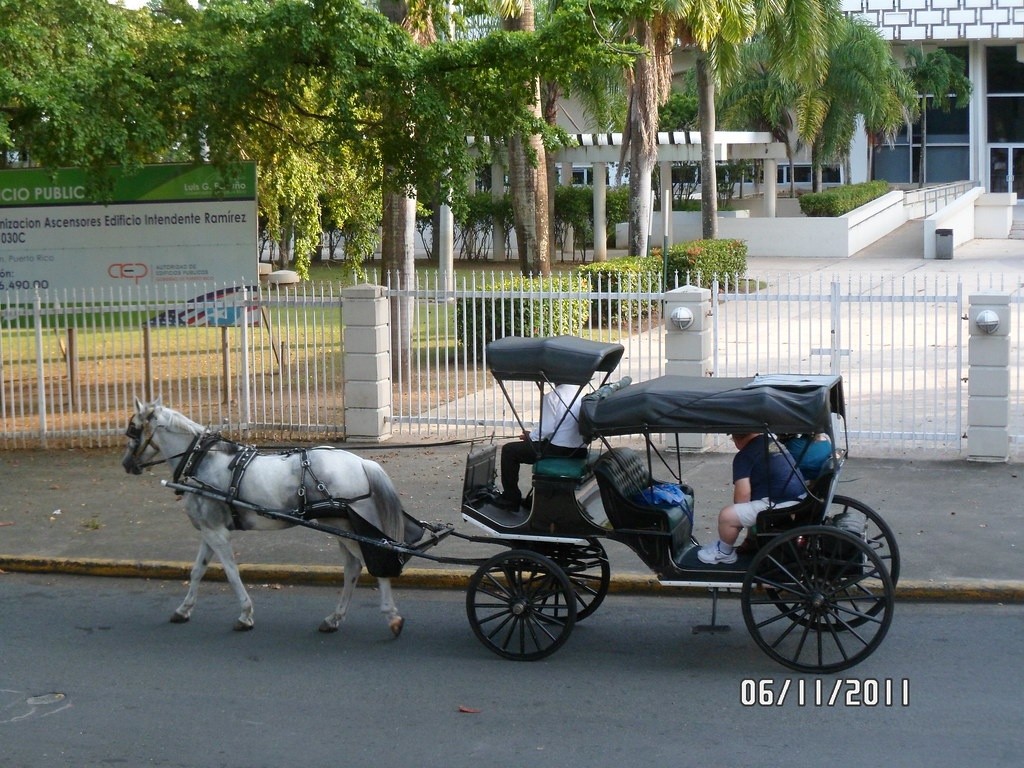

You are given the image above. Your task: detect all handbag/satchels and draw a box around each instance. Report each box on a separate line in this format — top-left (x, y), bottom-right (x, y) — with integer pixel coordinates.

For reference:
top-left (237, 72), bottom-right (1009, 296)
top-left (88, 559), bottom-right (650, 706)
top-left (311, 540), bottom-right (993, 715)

top-left (635, 482), bottom-right (694, 527)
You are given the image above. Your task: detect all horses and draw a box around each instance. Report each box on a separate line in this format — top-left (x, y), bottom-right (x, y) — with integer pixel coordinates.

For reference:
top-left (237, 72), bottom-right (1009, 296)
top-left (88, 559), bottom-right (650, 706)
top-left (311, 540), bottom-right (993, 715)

top-left (122, 393), bottom-right (404, 637)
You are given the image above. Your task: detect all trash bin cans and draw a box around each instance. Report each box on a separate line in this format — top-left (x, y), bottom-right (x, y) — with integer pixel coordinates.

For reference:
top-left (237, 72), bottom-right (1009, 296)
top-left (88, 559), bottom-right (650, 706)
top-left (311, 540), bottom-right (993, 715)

top-left (936, 228), bottom-right (953, 259)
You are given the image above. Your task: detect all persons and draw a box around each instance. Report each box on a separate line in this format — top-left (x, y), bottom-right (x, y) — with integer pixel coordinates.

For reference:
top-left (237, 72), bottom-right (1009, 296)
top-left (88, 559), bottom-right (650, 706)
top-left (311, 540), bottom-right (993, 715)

top-left (487, 383), bottom-right (589, 513)
top-left (696, 432), bottom-right (832, 564)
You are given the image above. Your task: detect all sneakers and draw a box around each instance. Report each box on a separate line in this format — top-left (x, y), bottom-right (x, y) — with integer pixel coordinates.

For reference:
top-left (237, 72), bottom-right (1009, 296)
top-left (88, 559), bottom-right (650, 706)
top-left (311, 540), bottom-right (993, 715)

top-left (697, 542), bottom-right (739, 566)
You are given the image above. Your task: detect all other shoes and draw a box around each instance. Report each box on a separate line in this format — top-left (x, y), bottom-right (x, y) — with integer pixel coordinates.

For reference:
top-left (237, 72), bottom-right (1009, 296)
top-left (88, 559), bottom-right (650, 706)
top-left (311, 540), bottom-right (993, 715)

top-left (488, 492), bottom-right (520, 512)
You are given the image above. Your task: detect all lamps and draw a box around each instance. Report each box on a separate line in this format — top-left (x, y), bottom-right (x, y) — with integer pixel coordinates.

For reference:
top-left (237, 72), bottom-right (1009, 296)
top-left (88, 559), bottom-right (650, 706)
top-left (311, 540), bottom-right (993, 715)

top-left (976, 310), bottom-right (1000, 333)
top-left (671, 307), bottom-right (693, 329)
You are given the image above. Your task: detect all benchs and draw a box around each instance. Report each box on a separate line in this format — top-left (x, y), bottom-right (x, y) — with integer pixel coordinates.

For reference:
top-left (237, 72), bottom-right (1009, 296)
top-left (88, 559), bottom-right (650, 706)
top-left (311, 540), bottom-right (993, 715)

top-left (749, 449), bottom-right (851, 560)
top-left (593, 445), bottom-right (695, 561)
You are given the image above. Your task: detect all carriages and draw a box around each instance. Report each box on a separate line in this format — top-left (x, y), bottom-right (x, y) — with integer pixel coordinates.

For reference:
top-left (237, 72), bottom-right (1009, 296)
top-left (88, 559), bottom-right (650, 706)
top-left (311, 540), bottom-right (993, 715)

top-left (121, 338), bottom-right (903, 676)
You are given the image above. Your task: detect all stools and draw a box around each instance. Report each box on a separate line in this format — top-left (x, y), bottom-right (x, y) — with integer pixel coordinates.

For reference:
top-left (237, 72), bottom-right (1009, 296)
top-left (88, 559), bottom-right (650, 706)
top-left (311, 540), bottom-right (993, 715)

top-left (532, 448), bottom-right (600, 481)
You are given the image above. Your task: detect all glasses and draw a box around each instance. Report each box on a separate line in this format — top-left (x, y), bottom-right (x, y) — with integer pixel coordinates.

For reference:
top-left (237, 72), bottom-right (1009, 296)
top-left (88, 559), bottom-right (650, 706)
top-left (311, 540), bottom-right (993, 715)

top-left (730, 434), bottom-right (741, 441)
